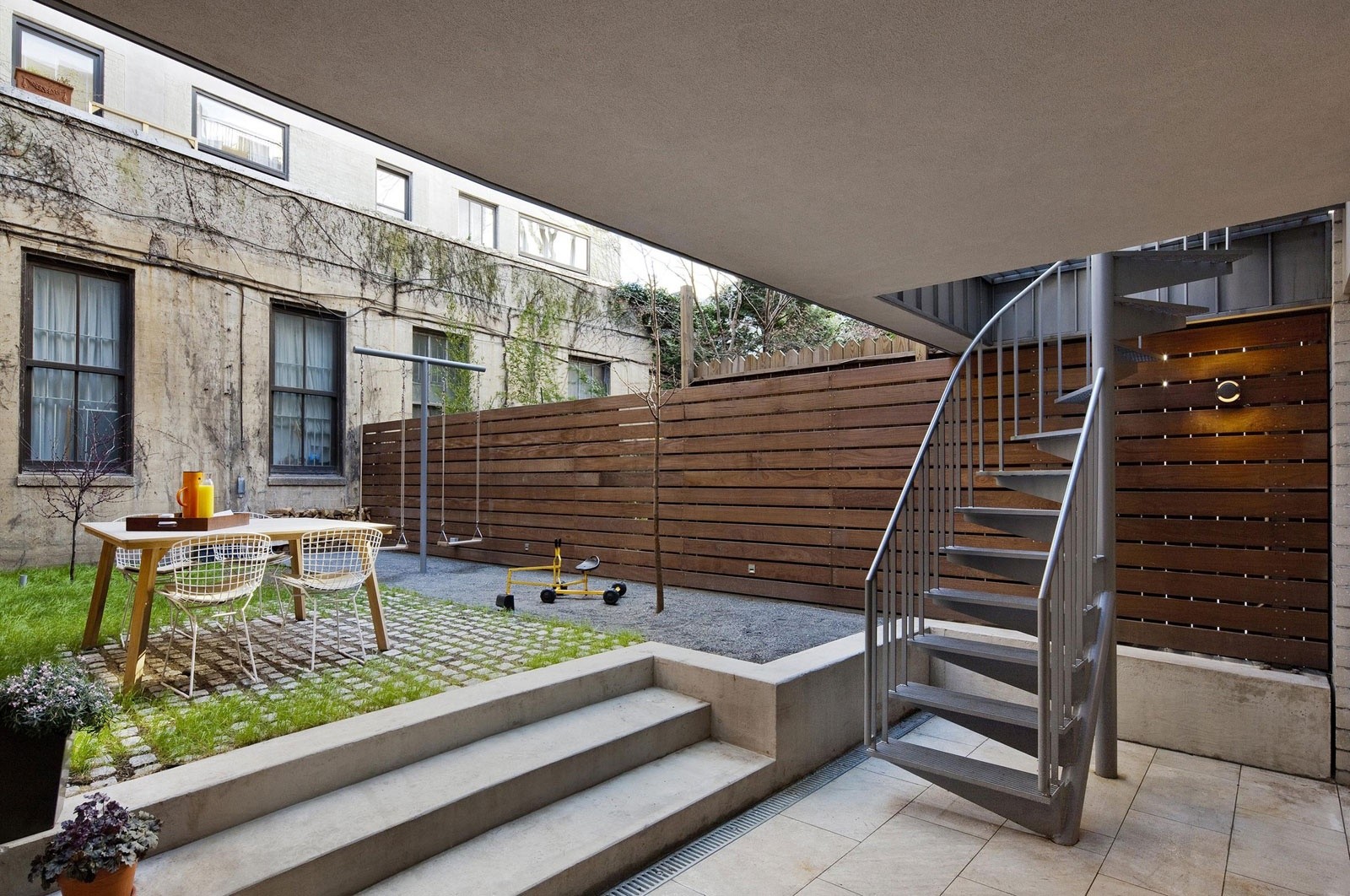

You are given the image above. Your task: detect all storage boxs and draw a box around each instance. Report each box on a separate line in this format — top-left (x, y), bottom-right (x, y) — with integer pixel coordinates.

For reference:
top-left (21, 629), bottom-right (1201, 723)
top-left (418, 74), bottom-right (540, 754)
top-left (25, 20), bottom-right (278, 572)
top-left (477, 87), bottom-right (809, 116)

top-left (126, 512), bottom-right (248, 531)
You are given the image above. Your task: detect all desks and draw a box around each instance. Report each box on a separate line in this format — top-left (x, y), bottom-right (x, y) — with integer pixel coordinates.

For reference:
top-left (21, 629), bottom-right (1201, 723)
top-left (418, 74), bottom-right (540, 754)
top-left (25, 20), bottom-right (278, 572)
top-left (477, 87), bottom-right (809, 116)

top-left (78, 516), bottom-right (395, 699)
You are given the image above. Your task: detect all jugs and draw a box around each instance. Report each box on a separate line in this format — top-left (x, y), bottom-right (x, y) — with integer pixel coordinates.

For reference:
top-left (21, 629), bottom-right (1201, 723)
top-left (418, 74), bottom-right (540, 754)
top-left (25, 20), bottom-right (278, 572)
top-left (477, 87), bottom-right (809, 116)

top-left (177, 470), bottom-right (204, 518)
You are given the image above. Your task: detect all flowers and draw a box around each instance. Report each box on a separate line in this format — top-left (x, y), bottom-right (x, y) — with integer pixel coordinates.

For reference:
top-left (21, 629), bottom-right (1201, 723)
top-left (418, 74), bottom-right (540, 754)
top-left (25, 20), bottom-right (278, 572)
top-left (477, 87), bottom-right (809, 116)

top-left (0, 657), bottom-right (121, 736)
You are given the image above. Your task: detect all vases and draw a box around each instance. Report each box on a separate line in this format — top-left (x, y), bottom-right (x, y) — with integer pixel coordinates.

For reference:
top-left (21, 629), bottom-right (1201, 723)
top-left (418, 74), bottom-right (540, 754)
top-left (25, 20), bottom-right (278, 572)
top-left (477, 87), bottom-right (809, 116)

top-left (0, 729), bottom-right (72, 846)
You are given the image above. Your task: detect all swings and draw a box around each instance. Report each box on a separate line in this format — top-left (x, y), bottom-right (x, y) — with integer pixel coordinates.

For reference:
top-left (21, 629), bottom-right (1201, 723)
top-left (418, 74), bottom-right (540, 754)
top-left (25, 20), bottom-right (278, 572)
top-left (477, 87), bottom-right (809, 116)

top-left (358, 355), bottom-right (410, 551)
top-left (436, 365), bottom-right (483, 548)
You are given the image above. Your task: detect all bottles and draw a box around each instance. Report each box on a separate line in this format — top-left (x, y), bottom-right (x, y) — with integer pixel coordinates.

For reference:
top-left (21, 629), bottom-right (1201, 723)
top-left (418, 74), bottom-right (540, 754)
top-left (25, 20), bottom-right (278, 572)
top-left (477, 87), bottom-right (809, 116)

top-left (196, 473), bottom-right (214, 519)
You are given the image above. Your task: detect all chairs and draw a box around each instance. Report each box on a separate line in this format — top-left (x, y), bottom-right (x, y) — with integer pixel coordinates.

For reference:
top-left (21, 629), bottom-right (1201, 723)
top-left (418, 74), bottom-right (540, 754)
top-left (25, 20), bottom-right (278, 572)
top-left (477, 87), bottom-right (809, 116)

top-left (109, 513), bottom-right (198, 650)
top-left (269, 526), bottom-right (384, 674)
top-left (154, 532), bottom-right (272, 700)
top-left (213, 512), bottom-right (294, 638)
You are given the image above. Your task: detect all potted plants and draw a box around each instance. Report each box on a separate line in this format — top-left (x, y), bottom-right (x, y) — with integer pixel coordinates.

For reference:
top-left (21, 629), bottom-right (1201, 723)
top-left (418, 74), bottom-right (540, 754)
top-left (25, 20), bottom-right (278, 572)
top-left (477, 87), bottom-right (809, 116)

top-left (26, 790), bottom-right (164, 896)
